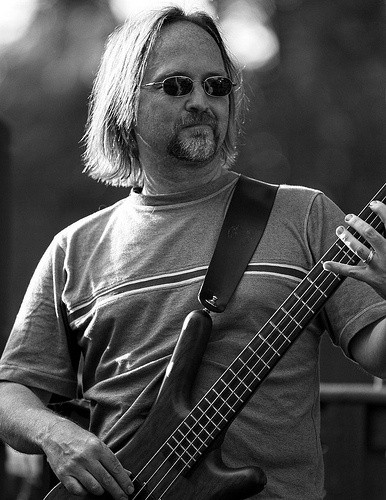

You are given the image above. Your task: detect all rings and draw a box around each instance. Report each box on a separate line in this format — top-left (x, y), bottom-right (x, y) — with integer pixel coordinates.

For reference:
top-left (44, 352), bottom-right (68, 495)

top-left (361, 250), bottom-right (374, 264)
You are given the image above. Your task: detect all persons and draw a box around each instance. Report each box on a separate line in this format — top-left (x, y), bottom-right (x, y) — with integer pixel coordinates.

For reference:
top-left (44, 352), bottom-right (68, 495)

top-left (0, 4), bottom-right (386, 500)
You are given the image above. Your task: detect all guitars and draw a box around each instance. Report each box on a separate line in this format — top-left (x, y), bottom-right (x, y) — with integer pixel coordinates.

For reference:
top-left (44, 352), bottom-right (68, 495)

top-left (42, 180), bottom-right (386, 500)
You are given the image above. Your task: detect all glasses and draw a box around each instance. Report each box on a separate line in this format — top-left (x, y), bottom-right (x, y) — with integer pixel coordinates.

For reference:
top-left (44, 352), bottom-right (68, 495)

top-left (137, 76), bottom-right (238, 98)
